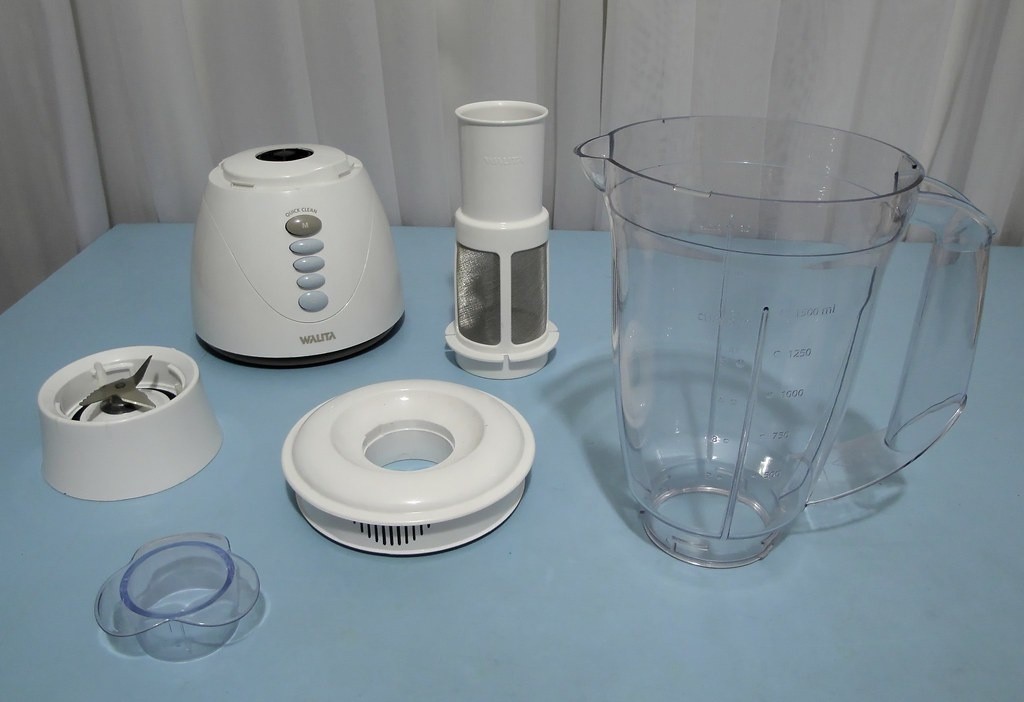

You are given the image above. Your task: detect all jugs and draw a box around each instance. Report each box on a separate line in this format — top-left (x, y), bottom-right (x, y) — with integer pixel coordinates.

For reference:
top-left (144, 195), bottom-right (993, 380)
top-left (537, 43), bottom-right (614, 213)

top-left (572, 115), bottom-right (994, 571)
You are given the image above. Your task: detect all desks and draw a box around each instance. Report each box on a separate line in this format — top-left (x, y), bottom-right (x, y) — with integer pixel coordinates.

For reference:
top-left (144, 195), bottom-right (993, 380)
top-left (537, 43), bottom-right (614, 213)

top-left (0, 220), bottom-right (1024, 702)
